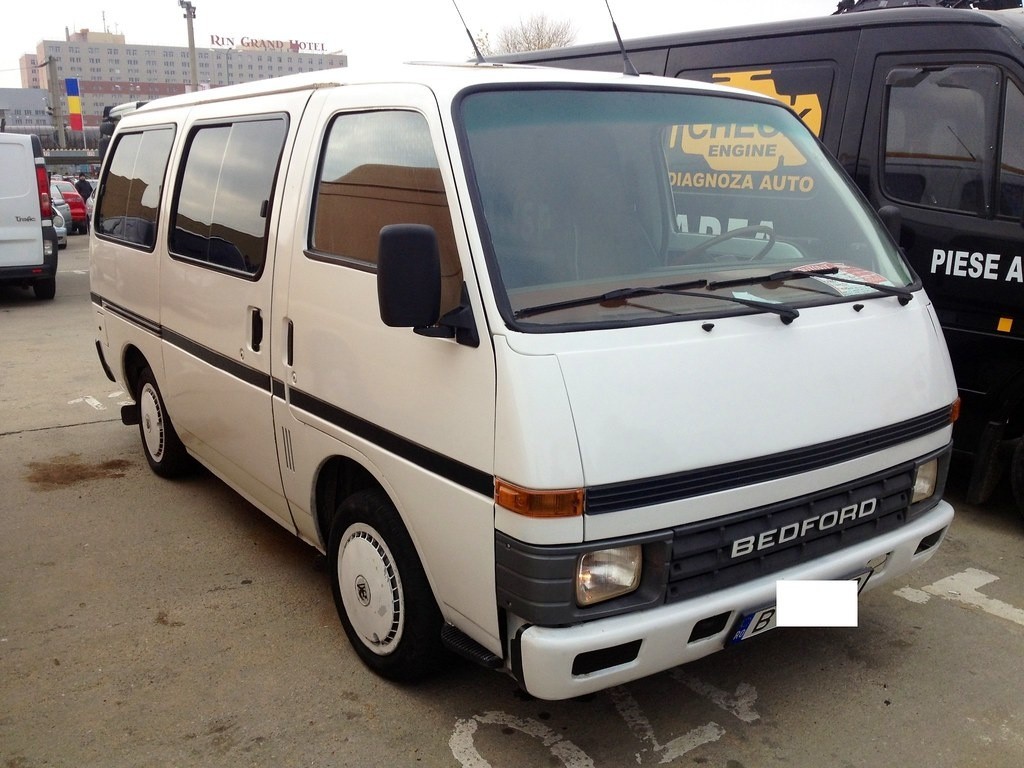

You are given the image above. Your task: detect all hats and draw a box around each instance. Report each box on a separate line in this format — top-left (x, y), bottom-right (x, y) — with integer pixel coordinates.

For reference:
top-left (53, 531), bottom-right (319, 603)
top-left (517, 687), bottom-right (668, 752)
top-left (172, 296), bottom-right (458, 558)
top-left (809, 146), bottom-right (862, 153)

top-left (79, 172), bottom-right (85, 176)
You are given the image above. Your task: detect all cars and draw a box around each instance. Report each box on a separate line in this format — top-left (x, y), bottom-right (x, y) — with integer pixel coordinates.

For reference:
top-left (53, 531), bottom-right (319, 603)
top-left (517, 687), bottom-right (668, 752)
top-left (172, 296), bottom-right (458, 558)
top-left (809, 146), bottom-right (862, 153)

top-left (51, 180), bottom-right (88, 234)
top-left (51, 205), bottom-right (67, 249)
top-left (99, 215), bottom-right (247, 274)
top-left (50, 184), bottom-right (72, 235)
top-left (85, 188), bottom-right (97, 234)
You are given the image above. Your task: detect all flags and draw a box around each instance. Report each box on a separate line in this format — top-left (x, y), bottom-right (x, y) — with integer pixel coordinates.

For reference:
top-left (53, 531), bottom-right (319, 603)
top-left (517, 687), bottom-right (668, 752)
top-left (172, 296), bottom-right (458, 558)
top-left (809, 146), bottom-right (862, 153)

top-left (65, 77), bottom-right (82, 132)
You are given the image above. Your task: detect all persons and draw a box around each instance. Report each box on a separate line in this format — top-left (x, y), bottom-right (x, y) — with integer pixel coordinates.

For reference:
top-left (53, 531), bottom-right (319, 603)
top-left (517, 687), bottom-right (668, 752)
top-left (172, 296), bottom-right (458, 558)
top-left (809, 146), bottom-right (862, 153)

top-left (74, 172), bottom-right (92, 205)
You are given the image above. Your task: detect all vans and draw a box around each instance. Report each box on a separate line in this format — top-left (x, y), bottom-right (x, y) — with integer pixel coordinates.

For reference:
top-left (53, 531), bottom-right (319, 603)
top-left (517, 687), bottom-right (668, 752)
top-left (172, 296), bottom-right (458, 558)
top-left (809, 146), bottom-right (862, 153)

top-left (472, 1), bottom-right (1024, 497)
top-left (0, 132), bottom-right (58, 300)
top-left (88, 61), bottom-right (961, 684)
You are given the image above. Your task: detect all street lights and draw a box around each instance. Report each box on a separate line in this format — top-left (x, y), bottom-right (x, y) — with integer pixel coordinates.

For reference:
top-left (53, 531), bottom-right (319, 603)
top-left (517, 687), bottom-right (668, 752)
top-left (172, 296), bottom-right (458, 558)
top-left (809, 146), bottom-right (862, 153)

top-left (177, 0), bottom-right (199, 92)
